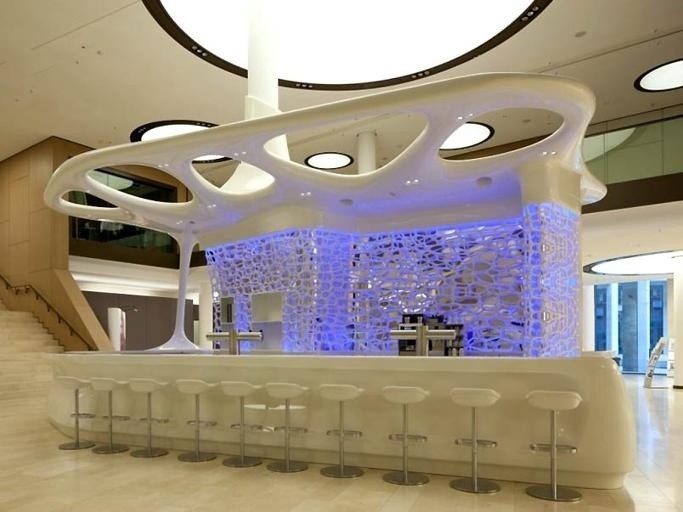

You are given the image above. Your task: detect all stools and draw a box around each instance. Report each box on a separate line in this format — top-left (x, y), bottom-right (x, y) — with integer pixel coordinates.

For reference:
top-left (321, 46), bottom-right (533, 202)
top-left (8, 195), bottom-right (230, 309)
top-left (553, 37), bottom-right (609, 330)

top-left (128, 378), bottom-right (169, 459)
top-left (220, 381), bottom-right (263, 468)
top-left (450, 388), bottom-right (502, 494)
top-left (318, 385), bottom-right (367, 479)
top-left (57, 375), bottom-right (94, 451)
top-left (526, 390), bottom-right (585, 503)
top-left (89, 377), bottom-right (130, 454)
top-left (266, 383), bottom-right (310, 474)
top-left (174, 379), bottom-right (217, 464)
top-left (382, 385), bottom-right (431, 487)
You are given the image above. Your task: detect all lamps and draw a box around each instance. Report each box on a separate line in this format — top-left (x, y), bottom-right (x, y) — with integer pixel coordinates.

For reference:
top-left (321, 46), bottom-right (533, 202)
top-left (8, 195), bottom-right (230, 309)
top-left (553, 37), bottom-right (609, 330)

top-left (304, 151), bottom-right (353, 170)
top-left (634, 58), bottom-right (683, 93)
top-left (130, 120), bottom-right (233, 164)
top-left (439, 121), bottom-right (495, 151)
top-left (142, 0), bottom-right (554, 91)
top-left (583, 249), bottom-right (683, 276)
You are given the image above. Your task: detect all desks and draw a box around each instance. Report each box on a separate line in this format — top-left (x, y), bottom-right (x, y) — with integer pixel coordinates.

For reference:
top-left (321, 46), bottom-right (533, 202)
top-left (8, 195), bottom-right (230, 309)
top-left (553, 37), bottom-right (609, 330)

top-left (43, 352), bottom-right (636, 489)
